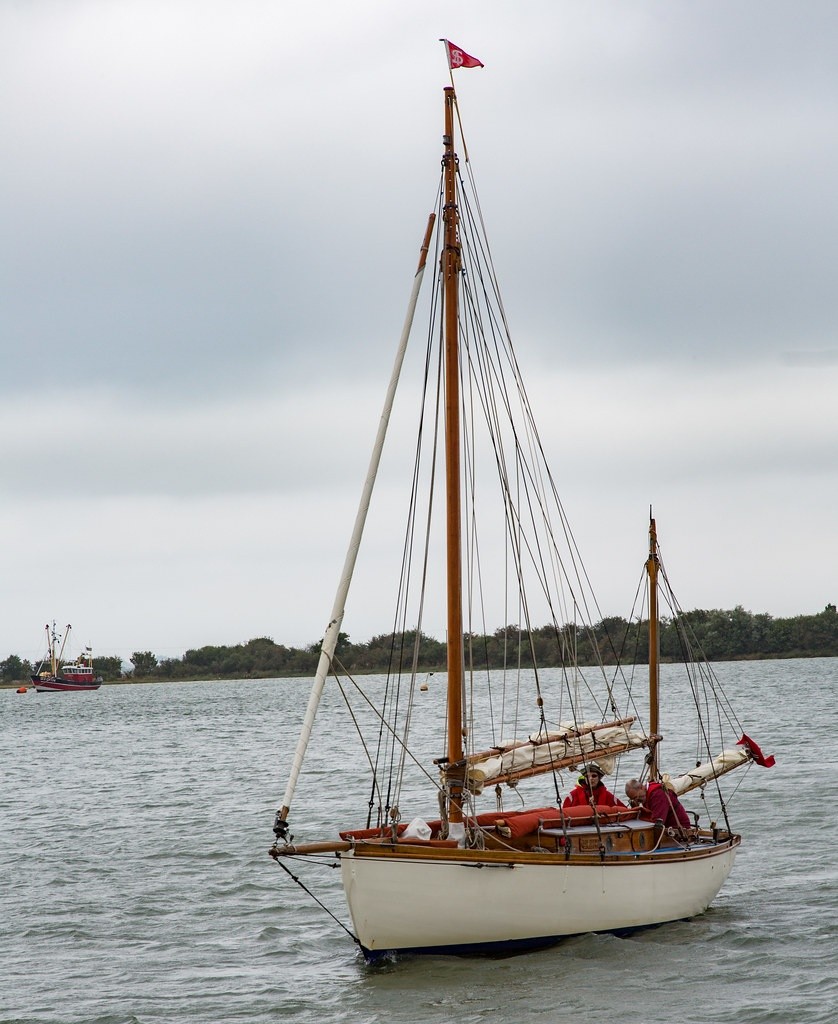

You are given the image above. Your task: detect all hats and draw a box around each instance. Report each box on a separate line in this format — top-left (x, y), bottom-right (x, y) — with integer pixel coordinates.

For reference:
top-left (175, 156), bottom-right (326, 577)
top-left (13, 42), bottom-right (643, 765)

top-left (580, 764), bottom-right (605, 777)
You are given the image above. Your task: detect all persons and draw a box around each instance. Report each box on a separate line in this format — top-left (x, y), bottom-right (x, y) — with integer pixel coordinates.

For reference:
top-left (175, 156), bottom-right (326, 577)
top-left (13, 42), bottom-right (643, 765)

top-left (563, 764), bottom-right (626, 809)
top-left (626, 779), bottom-right (690, 828)
top-left (84, 660), bottom-right (87, 667)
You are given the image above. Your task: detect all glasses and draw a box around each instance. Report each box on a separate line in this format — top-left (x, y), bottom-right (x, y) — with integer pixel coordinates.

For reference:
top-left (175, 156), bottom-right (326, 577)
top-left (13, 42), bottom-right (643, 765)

top-left (584, 774), bottom-right (597, 778)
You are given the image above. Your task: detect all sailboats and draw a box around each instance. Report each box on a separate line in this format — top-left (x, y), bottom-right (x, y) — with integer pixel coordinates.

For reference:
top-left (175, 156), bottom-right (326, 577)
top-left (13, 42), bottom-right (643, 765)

top-left (29, 619), bottom-right (103, 693)
top-left (266, 84), bottom-right (774, 959)
